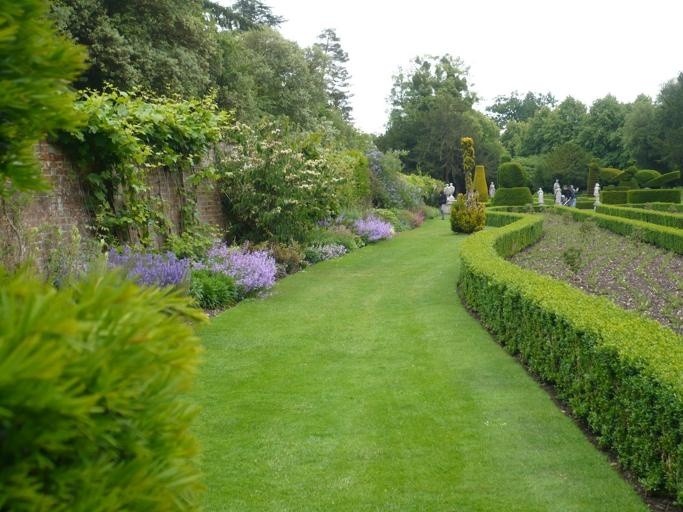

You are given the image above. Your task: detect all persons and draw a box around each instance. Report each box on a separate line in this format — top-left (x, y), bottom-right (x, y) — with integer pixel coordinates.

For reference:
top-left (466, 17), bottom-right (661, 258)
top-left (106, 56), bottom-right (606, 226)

top-left (555, 184), bottom-right (562, 205)
top-left (554, 178), bottom-right (560, 196)
top-left (562, 184), bottom-right (575, 209)
top-left (537, 187), bottom-right (545, 204)
top-left (437, 190), bottom-right (447, 220)
top-left (593, 180), bottom-right (602, 208)
top-left (489, 181), bottom-right (496, 199)
top-left (442, 183), bottom-right (449, 204)
top-left (447, 181), bottom-right (457, 204)
